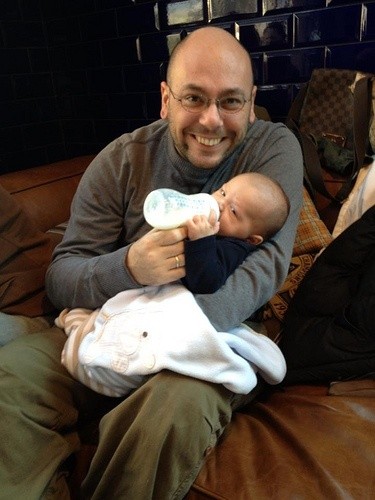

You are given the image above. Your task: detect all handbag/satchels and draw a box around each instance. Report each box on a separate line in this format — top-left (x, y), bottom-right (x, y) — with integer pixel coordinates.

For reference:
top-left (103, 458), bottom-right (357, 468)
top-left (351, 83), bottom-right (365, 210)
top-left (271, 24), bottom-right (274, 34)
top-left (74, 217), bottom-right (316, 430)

top-left (299, 69), bottom-right (369, 164)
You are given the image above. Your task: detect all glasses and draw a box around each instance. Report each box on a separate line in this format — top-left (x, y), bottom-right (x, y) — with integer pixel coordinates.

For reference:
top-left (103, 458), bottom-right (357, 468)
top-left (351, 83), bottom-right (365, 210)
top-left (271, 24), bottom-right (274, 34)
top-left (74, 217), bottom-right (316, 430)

top-left (168, 87), bottom-right (253, 114)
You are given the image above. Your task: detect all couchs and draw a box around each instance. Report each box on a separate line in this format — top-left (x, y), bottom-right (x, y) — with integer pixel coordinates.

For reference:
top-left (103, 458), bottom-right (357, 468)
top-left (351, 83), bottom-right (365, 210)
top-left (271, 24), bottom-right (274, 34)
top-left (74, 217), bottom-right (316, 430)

top-left (0, 106), bottom-right (374, 500)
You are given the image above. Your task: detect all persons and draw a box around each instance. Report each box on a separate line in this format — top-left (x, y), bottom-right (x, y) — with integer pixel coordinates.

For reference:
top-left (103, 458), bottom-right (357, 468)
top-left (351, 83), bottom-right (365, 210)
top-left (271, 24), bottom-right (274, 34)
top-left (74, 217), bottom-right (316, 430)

top-left (1, 26), bottom-right (302, 500)
top-left (182, 172), bottom-right (289, 294)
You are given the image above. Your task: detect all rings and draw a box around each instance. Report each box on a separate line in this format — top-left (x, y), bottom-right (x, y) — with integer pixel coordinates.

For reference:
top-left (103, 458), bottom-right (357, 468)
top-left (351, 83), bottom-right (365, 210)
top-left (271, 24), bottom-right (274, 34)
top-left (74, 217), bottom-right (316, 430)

top-left (174, 256), bottom-right (182, 269)
